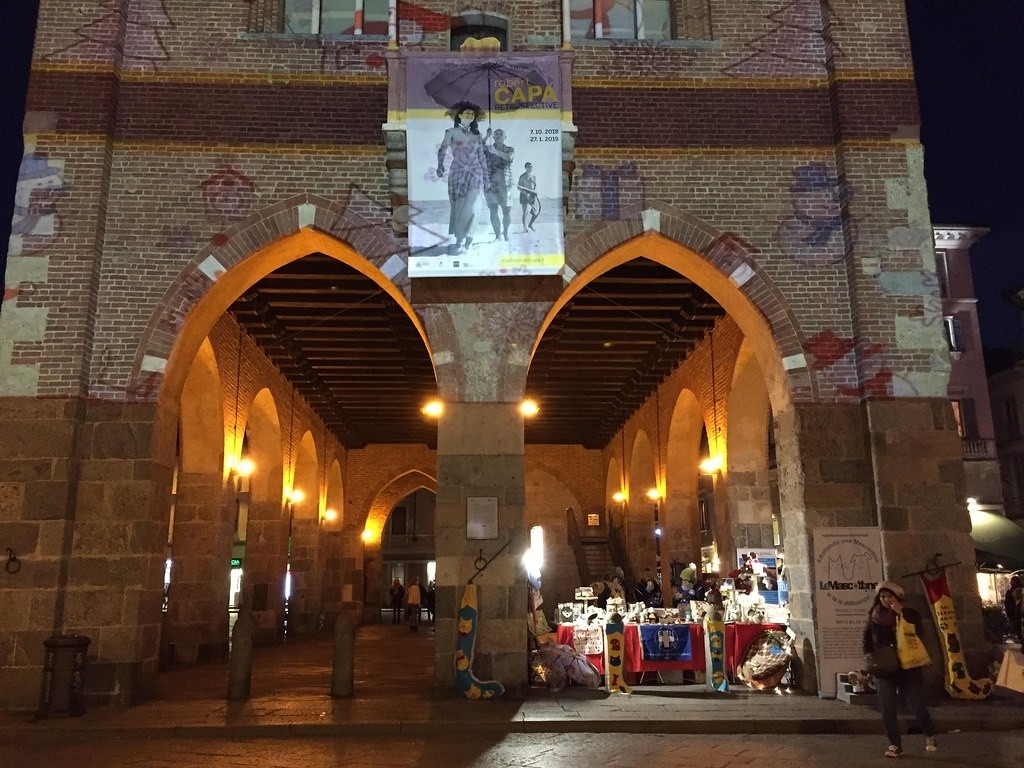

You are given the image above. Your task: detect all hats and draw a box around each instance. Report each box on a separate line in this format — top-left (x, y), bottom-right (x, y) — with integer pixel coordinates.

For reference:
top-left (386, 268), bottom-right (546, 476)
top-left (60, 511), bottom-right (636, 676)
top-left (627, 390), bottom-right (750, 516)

top-left (680, 567), bottom-right (694, 581)
top-left (875, 580), bottom-right (905, 598)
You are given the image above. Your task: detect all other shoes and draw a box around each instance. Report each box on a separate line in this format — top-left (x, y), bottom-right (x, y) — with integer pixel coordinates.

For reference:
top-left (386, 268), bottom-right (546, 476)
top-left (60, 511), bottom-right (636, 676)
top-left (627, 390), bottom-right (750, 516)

top-left (925, 736), bottom-right (937, 751)
top-left (392, 620), bottom-right (396, 624)
top-left (885, 744), bottom-right (905, 757)
top-left (397, 621), bottom-right (400, 624)
top-left (860, 669), bottom-right (877, 693)
top-left (847, 670), bottom-right (865, 693)
top-left (411, 628), bottom-right (417, 632)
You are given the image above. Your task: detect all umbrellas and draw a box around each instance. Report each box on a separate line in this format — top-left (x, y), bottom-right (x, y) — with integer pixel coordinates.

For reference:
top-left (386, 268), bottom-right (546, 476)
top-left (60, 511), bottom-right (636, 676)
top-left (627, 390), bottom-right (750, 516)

top-left (426, 58), bottom-right (549, 136)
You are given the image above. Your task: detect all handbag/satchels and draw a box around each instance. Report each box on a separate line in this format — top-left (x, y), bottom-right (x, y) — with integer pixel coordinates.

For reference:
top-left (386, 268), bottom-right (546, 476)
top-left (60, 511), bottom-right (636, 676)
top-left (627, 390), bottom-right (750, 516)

top-left (895, 612), bottom-right (930, 670)
top-left (996, 649), bottom-right (1024, 693)
top-left (861, 656), bottom-right (894, 680)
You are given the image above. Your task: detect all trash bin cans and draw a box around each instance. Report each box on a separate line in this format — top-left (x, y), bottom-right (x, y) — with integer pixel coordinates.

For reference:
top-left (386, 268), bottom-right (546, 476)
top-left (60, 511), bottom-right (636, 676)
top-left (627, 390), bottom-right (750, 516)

top-left (42, 634), bottom-right (91, 719)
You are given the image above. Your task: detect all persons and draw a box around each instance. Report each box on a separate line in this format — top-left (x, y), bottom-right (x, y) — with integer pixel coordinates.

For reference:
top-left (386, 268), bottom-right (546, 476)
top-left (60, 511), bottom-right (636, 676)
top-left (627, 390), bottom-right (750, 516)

top-left (590, 567), bottom-right (725, 610)
top-left (1005, 575), bottom-right (1024, 649)
top-left (436, 102), bottom-right (541, 253)
top-left (863, 580), bottom-right (936, 757)
top-left (389, 577), bottom-right (435, 631)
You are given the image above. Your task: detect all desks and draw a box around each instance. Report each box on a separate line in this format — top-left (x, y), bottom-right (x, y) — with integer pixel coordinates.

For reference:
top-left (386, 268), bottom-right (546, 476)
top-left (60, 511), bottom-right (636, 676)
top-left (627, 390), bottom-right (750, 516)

top-left (557, 622), bottom-right (783, 684)
